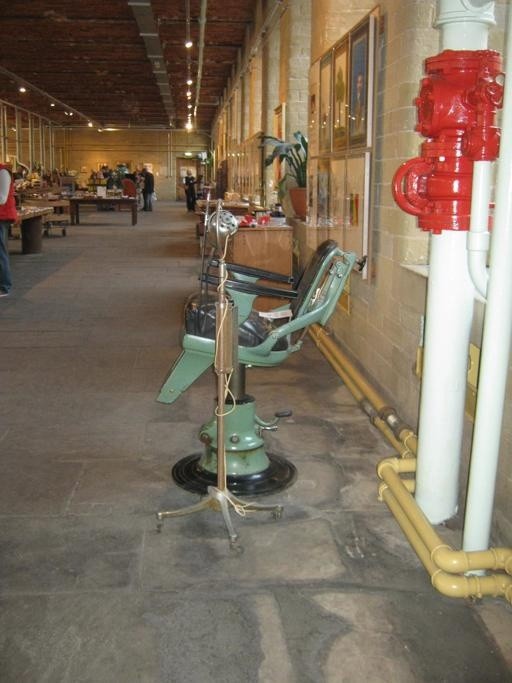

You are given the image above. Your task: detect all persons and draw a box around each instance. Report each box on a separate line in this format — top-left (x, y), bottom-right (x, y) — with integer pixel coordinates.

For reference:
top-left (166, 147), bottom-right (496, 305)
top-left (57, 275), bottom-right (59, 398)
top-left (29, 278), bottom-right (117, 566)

top-left (0, 162), bottom-right (18, 296)
top-left (183, 170), bottom-right (197, 211)
top-left (91, 165), bottom-right (154, 212)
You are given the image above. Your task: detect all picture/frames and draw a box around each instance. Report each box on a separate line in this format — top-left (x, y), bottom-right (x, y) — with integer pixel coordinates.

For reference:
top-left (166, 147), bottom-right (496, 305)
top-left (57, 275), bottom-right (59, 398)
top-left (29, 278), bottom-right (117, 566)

top-left (306, 14), bottom-right (373, 280)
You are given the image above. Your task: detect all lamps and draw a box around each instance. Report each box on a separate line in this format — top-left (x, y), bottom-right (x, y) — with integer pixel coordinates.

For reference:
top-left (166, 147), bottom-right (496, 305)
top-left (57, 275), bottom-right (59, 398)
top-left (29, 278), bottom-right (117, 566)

top-left (155, 192), bottom-right (283, 556)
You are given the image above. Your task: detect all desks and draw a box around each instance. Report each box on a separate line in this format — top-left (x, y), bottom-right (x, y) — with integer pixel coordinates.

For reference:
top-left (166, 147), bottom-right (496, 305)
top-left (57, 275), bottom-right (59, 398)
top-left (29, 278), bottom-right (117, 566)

top-left (194, 199), bottom-right (249, 256)
top-left (225, 215), bottom-right (294, 313)
top-left (17, 188), bottom-right (144, 254)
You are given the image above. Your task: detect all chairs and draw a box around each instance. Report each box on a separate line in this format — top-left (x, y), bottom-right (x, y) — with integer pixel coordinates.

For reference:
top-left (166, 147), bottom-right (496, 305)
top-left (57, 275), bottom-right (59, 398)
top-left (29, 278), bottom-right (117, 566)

top-left (155, 237), bottom-right (357, 503)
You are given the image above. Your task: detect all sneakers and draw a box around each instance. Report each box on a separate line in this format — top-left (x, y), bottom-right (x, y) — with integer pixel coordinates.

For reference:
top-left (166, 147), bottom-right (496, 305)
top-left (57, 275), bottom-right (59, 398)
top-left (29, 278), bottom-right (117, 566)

top-left (0, 280), bottom-right (13, 298)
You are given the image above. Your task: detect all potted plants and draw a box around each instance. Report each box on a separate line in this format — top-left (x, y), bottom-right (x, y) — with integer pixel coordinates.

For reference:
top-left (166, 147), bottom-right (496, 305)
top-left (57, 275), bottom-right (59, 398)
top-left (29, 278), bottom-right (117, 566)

top-left (256, 131), bottom-right (308, 221)
